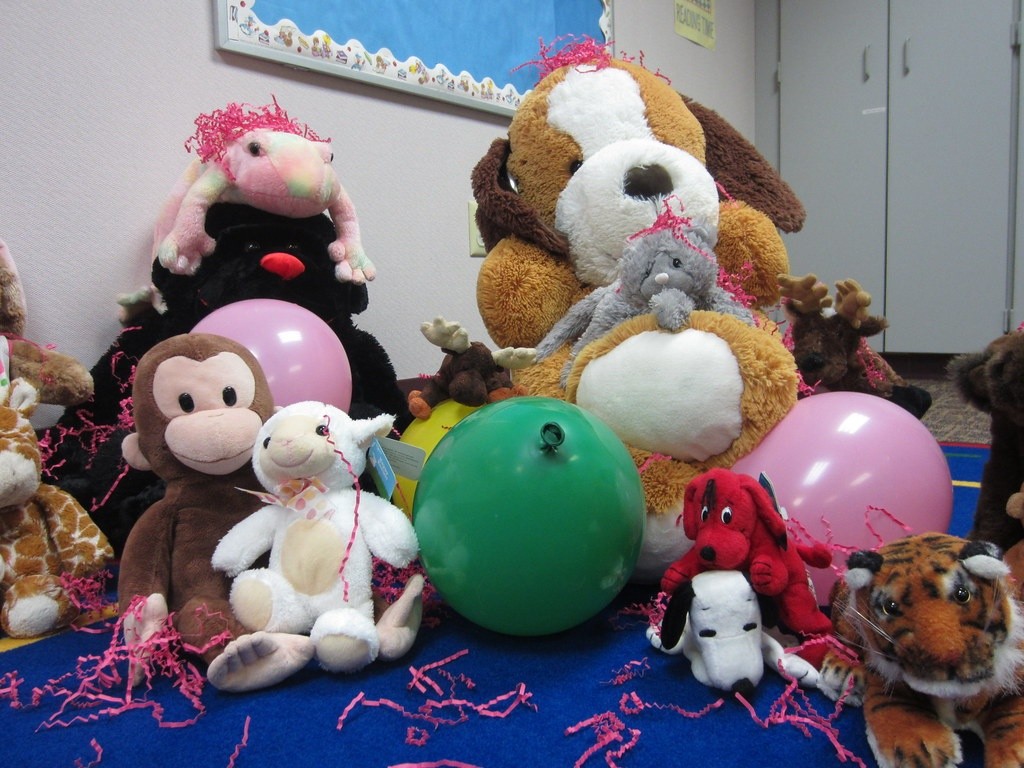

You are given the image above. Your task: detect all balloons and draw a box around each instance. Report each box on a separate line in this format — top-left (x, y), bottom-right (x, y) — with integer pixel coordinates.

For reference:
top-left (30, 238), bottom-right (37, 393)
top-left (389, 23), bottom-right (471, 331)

top-left (187, 297), bottom-right (351, 414)
top-left (730, 390), bottom-right (955, 609)
top-left (390, 397), bottom-right (487, 525)
top-left (411, 397), bottom-right (647, 638)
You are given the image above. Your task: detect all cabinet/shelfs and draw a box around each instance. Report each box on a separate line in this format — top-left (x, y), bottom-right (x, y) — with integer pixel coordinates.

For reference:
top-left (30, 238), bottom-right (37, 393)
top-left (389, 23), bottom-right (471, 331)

top-left (755, 0), bottom-right (1024, 353)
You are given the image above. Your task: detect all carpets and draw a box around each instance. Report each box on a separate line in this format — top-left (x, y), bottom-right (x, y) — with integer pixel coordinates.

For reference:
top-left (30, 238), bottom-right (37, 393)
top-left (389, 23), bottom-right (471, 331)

top-left (1, 442), bottom-right (1002, 768)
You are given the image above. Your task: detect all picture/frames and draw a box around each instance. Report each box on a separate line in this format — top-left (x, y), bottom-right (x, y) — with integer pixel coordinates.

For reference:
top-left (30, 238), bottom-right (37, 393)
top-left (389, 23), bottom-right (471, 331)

top-left (210, 0), bottom-right (614, 120)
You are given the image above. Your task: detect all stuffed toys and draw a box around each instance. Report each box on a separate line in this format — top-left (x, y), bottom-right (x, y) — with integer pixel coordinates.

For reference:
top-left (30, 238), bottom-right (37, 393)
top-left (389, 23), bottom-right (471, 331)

top-left (1, 30), bottom-right (1024, 768)
